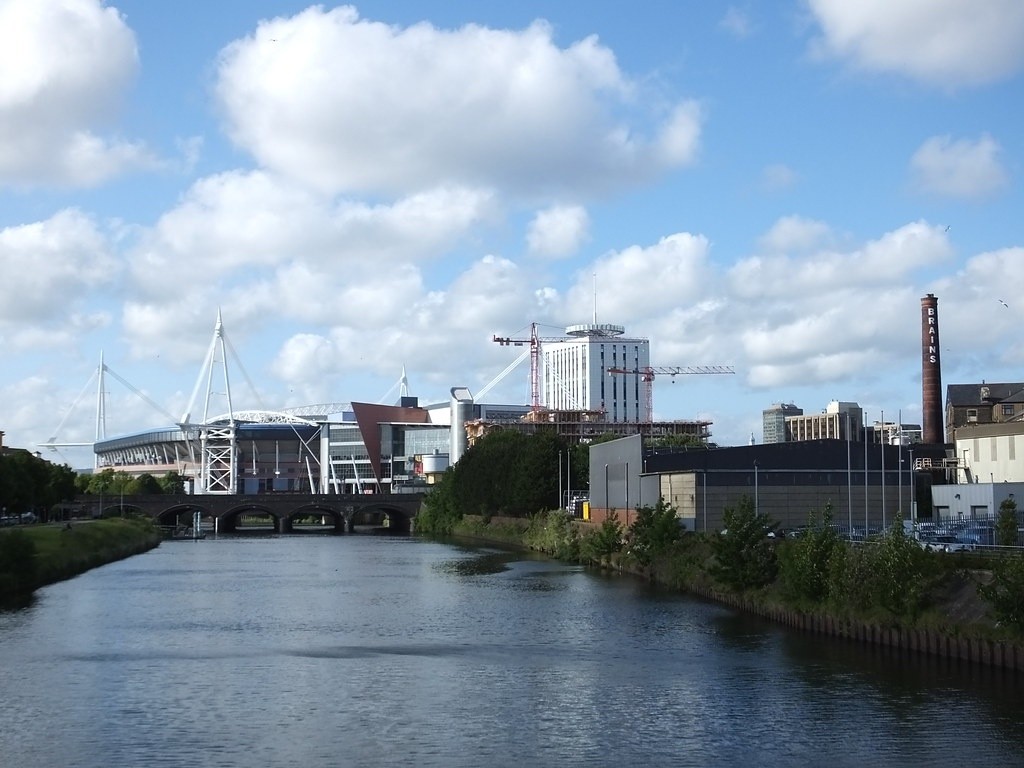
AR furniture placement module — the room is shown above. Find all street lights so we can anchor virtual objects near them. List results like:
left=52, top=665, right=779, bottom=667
left=121, top=483, right=126, bottom=515
left=99, top=482, right=110, bottom=519
left=753, top=456, right=760, bottom=517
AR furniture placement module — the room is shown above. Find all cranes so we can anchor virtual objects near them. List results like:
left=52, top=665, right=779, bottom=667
left=606, top=365, right=736, bottom=421
left=493, top=322, right=650, bottom=410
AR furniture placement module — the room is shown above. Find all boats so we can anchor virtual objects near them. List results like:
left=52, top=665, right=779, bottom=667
left=172, top=525, right=206, bottom=540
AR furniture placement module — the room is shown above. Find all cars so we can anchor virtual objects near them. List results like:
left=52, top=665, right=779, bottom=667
left=0, top=512, right=38, bottom=526
left=767, top=520, right=996, bottom=553
